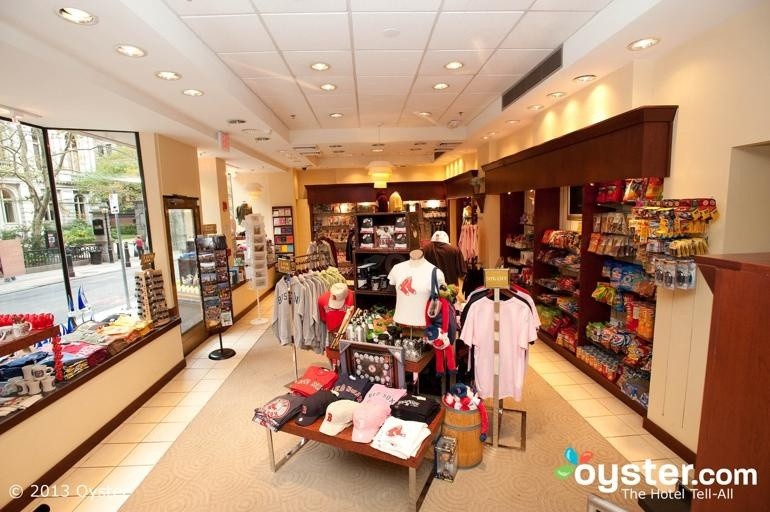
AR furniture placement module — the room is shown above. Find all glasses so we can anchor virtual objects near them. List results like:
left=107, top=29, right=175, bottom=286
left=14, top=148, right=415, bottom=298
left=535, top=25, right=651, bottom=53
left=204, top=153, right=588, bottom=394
left=134, top=273, right=171, bottom=325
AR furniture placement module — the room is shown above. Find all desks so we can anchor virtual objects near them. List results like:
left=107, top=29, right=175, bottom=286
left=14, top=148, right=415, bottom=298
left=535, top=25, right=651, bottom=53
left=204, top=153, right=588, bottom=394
left=263, top=385, right=446, bottom=511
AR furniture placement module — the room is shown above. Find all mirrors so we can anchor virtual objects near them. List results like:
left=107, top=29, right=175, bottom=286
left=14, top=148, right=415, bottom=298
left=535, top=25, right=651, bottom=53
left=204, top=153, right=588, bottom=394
left=165, top=194, right=205, bottom=335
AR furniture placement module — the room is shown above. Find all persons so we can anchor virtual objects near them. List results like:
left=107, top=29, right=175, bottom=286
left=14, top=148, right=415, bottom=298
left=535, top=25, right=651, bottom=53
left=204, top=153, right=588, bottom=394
left=133, top=235, right=144, bottom=259
left=387, top=250, right=448, bottom=327
left=419, top=230, right=466, bottom=302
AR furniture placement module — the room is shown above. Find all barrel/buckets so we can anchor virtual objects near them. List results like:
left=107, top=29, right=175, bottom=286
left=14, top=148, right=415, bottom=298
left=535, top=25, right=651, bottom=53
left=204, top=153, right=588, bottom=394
left=441, top=403, right=482, bottom=469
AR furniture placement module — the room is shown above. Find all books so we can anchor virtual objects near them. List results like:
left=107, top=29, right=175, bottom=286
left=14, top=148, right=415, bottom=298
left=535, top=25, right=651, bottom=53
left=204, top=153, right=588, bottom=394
left=272, top=207, right=294, bottom=272
left=197, top=234, right=233, bottom=330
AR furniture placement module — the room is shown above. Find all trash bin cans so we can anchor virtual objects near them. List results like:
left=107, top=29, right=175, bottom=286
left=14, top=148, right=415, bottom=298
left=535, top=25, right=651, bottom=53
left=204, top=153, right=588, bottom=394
left=90, top=250, right=101, bottom=264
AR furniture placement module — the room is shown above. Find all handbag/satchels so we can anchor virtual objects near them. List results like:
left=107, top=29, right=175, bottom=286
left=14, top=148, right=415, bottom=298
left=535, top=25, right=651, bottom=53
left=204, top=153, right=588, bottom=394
left=426, top=266, right=457, bottom=345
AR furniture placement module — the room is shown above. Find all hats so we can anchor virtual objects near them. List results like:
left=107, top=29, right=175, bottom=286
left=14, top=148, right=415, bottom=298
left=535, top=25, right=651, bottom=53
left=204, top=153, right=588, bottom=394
left=294, top=390, right=338, bottom=427
left=351, top=399, right=392, bottom=444
left=430, top=230, right=453, bottom=245
left=319, top=399, right=359, bottom=438
left=328, top=281, right=349, bottom=310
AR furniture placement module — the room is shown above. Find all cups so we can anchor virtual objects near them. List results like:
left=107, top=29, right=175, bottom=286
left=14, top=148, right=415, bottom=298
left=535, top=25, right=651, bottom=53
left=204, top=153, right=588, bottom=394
left=357, top=262, right=389, bottom=291
left=9, top=364, right=56, bottom=395
left=0, top=322, right=32, bottom=339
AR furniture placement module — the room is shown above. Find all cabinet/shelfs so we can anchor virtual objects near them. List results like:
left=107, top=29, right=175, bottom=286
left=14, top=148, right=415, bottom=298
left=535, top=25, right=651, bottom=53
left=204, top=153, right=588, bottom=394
left=504, top=242, right=582, bottom=356
left=313, top=213, right=352, bottom=253
left=354, top=212, right=421, bottom=315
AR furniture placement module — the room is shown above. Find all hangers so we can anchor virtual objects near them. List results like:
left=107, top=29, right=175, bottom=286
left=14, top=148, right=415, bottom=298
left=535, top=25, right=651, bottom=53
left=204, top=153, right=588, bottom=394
left=281, top=254, right=329, bottom=281
left=487, top=286, right=513, bottom=301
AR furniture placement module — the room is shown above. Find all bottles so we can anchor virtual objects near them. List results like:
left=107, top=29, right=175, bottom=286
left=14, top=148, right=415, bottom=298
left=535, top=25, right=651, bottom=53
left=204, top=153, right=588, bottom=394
left=347, top=311, right=389, bottom=346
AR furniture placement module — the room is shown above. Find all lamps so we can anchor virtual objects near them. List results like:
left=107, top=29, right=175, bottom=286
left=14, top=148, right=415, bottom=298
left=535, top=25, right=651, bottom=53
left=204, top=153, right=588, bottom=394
left=365, top=123, right=396, bottom=188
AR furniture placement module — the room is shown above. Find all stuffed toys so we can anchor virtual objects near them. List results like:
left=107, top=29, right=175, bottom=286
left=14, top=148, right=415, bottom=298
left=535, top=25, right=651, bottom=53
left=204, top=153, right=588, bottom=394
left=439, top=442, right=457, bottom=475
